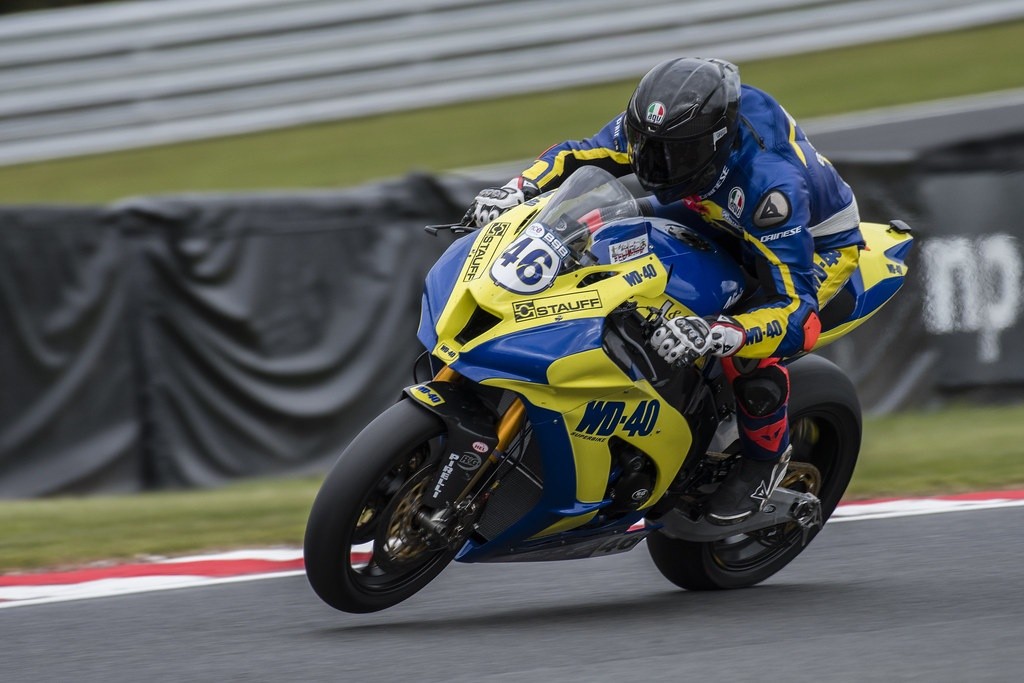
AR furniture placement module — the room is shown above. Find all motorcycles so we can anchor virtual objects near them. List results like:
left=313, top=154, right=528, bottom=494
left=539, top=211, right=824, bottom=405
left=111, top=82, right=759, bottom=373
left=301, top=163, right=913, bottom=614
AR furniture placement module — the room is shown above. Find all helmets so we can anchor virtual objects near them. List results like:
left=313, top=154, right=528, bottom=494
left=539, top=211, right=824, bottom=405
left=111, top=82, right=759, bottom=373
left=627, top=57, right=742, bottom=206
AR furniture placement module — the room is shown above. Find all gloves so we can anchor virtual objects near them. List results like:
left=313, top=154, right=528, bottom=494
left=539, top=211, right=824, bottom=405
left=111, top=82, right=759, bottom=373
left=460, top=176, right=542, bottom=229
left=650, top=314, right=746, bottom=369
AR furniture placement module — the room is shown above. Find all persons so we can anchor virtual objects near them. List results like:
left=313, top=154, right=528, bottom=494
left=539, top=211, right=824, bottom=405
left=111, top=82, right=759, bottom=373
left=458, top=56, right=867, bottom=527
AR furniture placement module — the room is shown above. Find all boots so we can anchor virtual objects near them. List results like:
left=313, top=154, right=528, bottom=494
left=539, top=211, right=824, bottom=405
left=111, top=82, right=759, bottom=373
left=704, top=401, right=792, bottom=526
